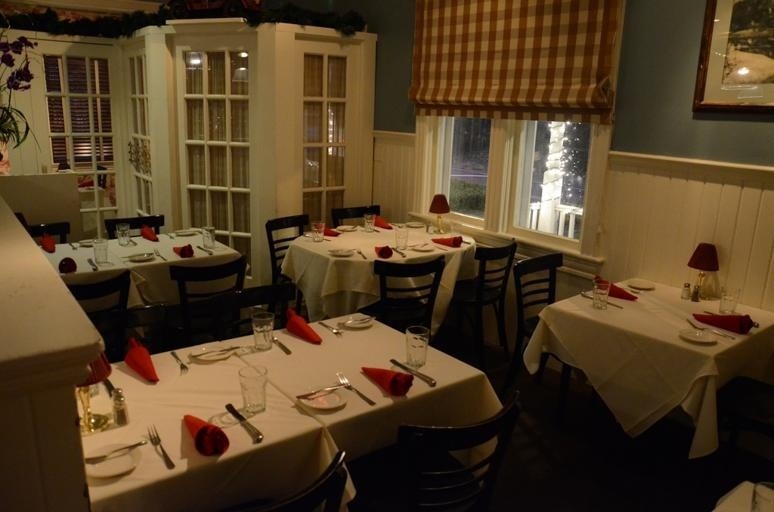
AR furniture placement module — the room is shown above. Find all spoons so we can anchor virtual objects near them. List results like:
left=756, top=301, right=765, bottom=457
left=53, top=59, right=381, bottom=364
left=68, top=241, right=77, bottom=250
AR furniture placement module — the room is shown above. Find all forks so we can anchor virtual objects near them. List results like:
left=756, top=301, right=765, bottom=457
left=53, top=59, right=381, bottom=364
left=172, top=351, right=189, bottom=372
left=319, top=321, right=344, bottom=334
left=147, top=424, right=175, bottom=469
left=336, top=372, right=376, bottom=406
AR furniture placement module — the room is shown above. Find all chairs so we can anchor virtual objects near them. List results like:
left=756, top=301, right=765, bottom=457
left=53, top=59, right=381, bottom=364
left=274, top=449, right=348, bottom=512
left=396, top=391, right=520, bottom=512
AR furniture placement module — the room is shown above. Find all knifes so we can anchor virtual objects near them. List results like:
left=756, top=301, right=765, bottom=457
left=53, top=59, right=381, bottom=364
left=85, top=440, right=147, bottom=462
left=87, top=259, right=99, bottom=271
left=271, top=334, right=291, bottom=354
left=581, top=291, right=623, bottom=310
left=303, top=233, right=332, bottom=241
left=103, top=376, right=116, bottom=398
left=295, top=385, right=344, bottom=400
left=225, top=404, right=264, bottom=442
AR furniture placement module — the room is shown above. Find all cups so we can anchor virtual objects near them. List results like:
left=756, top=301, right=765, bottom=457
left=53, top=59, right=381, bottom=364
left=239, top=365, right=268, bottom=413
left=251, top=314, right=274, bottom=351
left=237, top=313, right=437, bottom=416
left=719, top=286, right=741, bottom=315
left=93, top=222, right=216, bottom=265
left=592, top=279, right=612, bottom=309
left=311, top=214, right=409, bottom=250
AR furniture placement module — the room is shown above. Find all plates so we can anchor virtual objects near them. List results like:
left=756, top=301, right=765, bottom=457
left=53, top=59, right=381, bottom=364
left=78, top=229, right=197, bottom=263
left=678, top=327, right=718, bottom=345
left=327, top=221, right=434, bottom=260
left=300, top=385, right=348, bottom=410
left=85, top=443, right=141, bottom=478
left=628, top=279, right=655, bottom=289
left=336, top=315, right=374, bottom=330
left=190, top=342, right=234, bottom=361
left=79, top=413, right=110, bottom=437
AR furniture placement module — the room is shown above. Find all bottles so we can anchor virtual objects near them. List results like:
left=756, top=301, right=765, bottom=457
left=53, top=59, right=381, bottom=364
left=681, top=283, right=700, bottom=302
left=111, top=388, right=130, bottom=428
